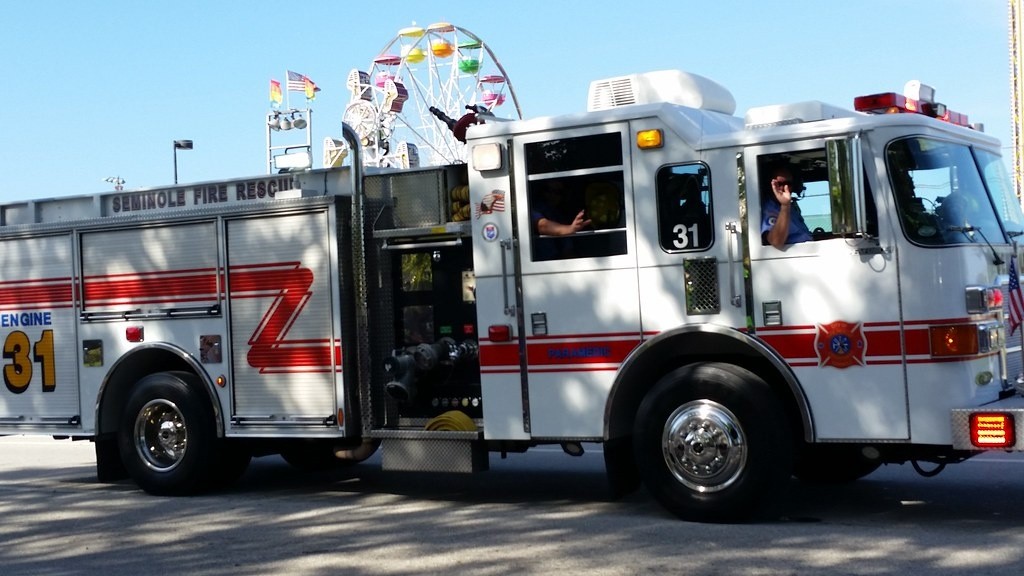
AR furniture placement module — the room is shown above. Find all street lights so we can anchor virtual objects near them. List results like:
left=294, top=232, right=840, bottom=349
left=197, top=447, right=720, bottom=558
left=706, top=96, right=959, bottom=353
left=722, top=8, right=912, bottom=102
left=172, top=138, right=194, bottom=184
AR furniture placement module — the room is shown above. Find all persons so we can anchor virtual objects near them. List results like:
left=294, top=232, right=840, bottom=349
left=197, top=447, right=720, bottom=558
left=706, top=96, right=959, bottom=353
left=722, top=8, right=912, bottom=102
left=529, top=170, right=592, bottom=261
left=760, top=166, right=814, bottom=249
left=658, top=175, right=707, bottom=248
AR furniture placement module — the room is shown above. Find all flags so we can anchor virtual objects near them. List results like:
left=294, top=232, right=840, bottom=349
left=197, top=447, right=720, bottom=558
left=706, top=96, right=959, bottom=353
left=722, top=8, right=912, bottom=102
left=1008, top=261, right=1024, bottom=336
left=288, top=70, right=321, bottom=91
left=270, top=80, right=283, bottom=105
left=304, top=77, right=315, bottom=99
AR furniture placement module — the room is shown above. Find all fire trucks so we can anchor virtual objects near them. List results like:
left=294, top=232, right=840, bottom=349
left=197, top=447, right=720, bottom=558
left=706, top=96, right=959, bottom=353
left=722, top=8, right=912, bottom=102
left=0, top=70, right=1024, bottom=525
